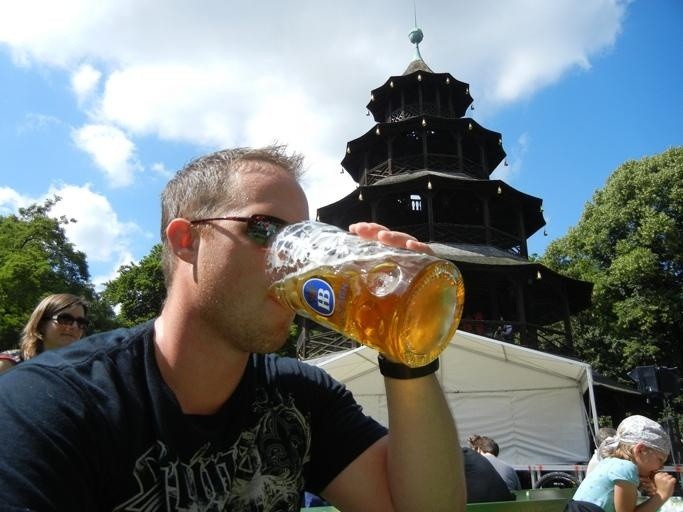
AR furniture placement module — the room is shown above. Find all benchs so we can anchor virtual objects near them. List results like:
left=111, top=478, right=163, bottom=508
left=300, top=484, right=581, bottom=512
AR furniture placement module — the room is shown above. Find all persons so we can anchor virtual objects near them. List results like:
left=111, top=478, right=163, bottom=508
left=561, top=414, right=677, bottom=511
left=582, top=425, right=618, bottom=477
left=0, top=295, right=87, bottom=372
left=466, top=433, right=521, bottom=490
left=0, top=142, right=468, bottom=512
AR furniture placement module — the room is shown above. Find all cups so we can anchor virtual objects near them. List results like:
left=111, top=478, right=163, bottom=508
left=262, top=217, right=464, bottom=370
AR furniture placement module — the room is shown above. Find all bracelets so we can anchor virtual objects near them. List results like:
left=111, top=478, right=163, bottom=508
left=376, top=352, right=439, bottom=381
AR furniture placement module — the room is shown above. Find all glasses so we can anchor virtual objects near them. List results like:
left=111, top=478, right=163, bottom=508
left=47, top=311, right=90, bottom=330
left=191, top=213, right=287, bottom=248
